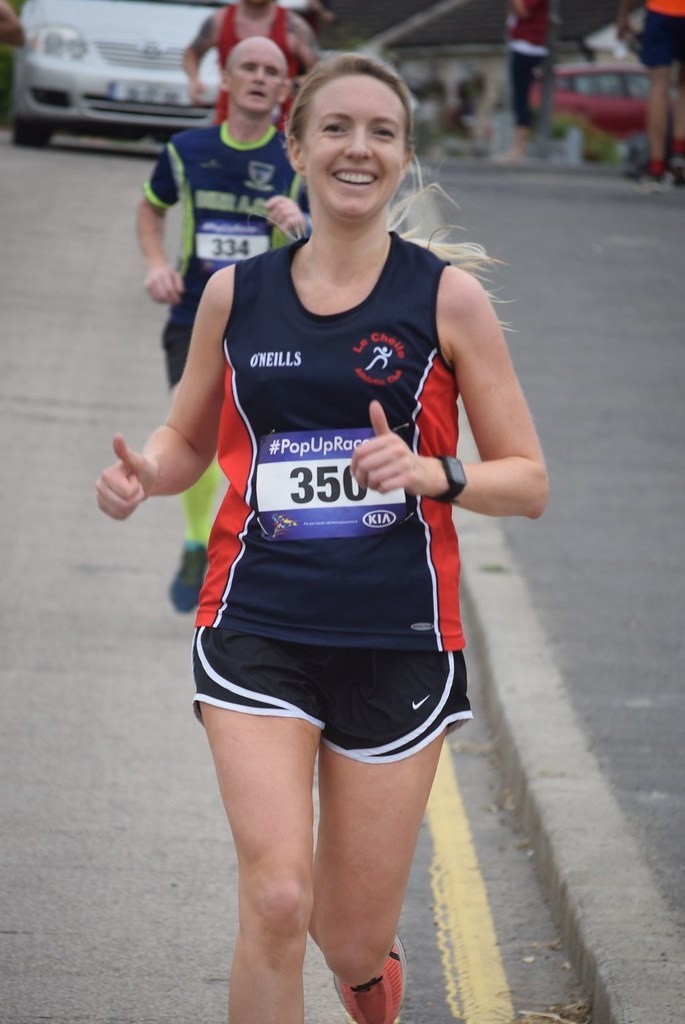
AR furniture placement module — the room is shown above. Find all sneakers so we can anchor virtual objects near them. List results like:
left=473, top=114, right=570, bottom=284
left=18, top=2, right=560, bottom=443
left=333, top=930, right=406, bottom=1024
left=170, top=542, right=208, bottom=613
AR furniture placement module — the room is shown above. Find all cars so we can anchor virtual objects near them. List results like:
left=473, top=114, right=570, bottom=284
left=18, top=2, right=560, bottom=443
left=10, top=0, right=237, bottom=148
left=527, top=62, right=684, bottom=180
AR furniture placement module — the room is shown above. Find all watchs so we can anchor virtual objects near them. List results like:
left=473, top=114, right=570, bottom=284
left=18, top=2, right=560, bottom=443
left=427, top=453, right=467, bottom=502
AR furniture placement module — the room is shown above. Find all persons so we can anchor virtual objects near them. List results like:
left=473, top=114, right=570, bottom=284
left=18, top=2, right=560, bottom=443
left=96, top=50, right=551, bottom=1024
left=0, top=0, right=26, bottom=119
left=183, top=0, right=315, bottom=134
left=505, top=0, right=552, bottom=152
left=134, top=35, right=310, bottom=612
left=616, top=0, right=685, bottom=186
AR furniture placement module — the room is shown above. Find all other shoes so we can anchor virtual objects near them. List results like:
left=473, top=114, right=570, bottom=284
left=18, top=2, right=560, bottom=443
left=671, top=155, right=685, bottom=183
